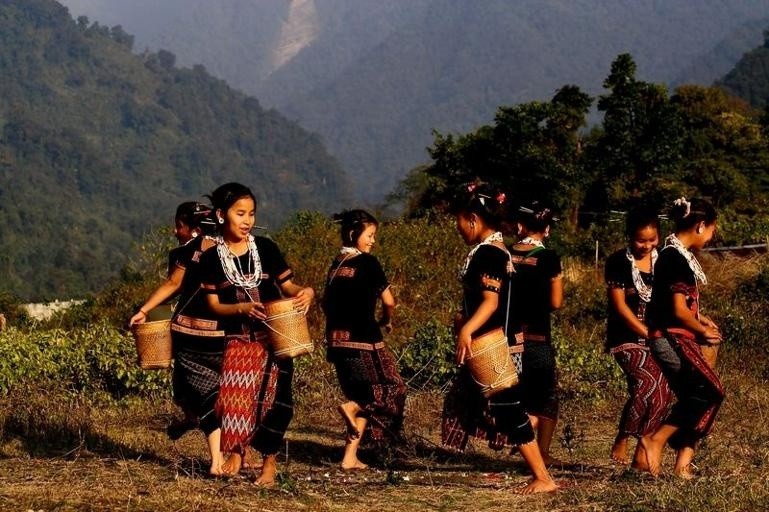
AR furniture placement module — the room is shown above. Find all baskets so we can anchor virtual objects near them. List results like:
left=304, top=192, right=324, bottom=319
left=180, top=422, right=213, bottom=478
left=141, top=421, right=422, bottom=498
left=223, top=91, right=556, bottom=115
left=465, top=327, right=519, bottom=398
left=262, top=296, right=315, bottom=359
left=700, top=345, right=718, bottom=370
left=132, top=319, right=172, bottom=370
